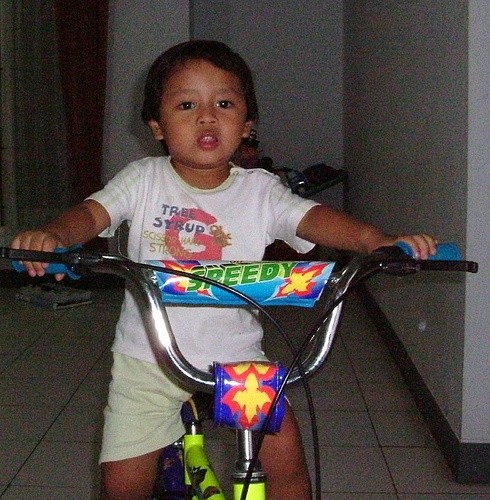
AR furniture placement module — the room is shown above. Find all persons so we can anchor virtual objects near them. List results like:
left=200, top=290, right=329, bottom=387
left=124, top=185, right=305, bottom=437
left=6, top=41, right=437, bottom=500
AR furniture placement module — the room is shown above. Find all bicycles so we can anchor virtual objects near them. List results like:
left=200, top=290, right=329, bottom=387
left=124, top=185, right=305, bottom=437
left=0, top=232, right=478, bottom=500
